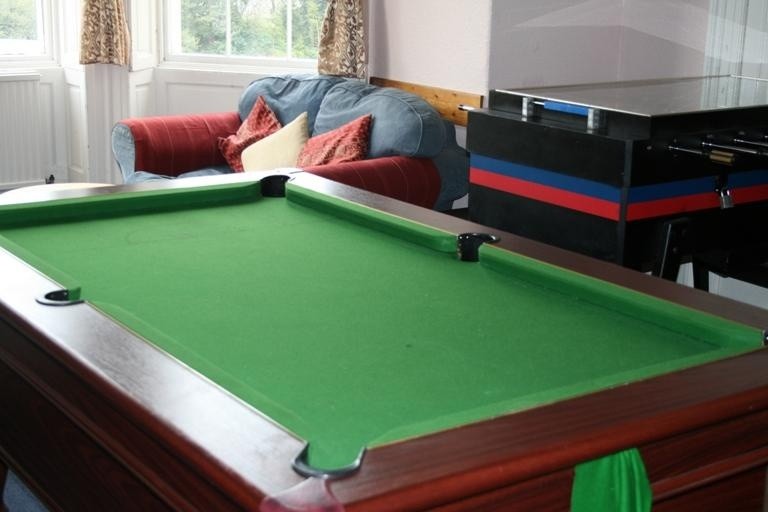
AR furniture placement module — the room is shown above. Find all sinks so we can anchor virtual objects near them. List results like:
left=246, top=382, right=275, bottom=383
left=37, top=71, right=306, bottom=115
left=110, top=73, right=468, bottom=211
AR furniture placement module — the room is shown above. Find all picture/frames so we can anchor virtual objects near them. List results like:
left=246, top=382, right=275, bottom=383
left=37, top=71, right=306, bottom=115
left=0, top=170, right=768, bottom=512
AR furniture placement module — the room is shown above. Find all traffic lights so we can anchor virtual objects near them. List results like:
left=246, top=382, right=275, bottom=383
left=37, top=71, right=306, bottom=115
left=216, top=95, right=373, bottom=175
